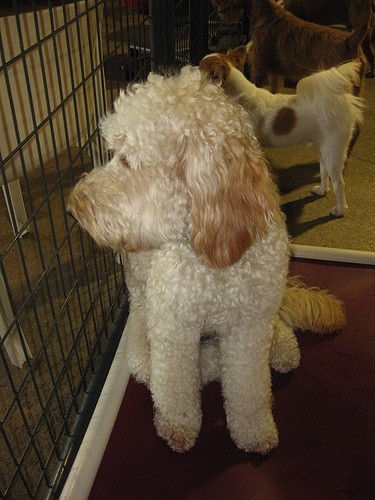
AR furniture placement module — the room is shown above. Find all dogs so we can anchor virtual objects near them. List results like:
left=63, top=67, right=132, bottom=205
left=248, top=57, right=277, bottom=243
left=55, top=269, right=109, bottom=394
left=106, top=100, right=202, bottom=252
left=195, top=45, right=368, bottom=216
left=217, top=0, right=372, bottom=158
left=65, top=65, right=347, bottom=455
